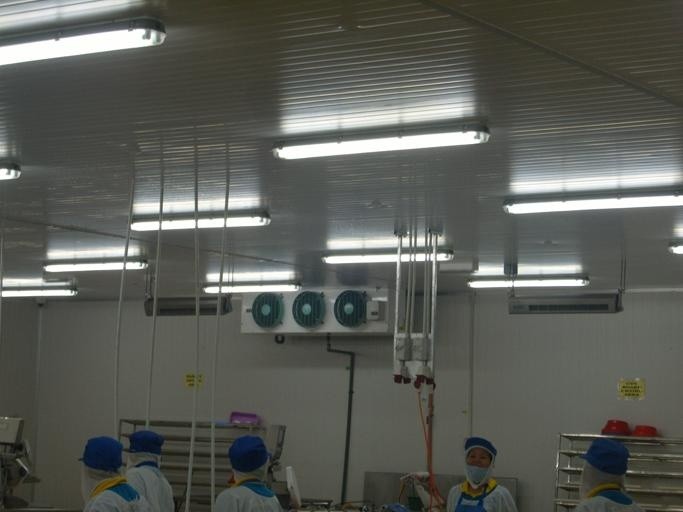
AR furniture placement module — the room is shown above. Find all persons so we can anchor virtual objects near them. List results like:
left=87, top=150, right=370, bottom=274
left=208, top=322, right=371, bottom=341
left=214, top=435, right=286, bottom=512
left=122, top=431, right=175, bottom=512
left=77, top=435, right=148, bottom=512
left=568, top=438, right=644, bottom=512
left=447, top=436, right=518, bottom=512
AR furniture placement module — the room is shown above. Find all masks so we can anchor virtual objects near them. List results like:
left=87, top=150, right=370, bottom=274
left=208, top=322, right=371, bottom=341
left=464, top=464, right=489, bottom=483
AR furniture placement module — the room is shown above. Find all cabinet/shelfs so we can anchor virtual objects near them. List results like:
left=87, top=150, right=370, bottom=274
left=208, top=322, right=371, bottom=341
left=552, top=430, right=682, bottom=512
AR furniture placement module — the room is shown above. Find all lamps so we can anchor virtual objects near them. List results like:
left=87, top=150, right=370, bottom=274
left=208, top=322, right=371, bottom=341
left=271, top=123, right=490, bottom=159
left=468, top=277, right=588, bottom=289
left=132, top=213, right=272, bottom=233
left=1, top=286, right=76, bottom=298
left=323, top=250, right=454, bottom=264
left=44, top=260, right=149, bottom=272
left=502, top=191, right=682, bottom=214
left=1, top=16, right=166, bottom=65
left=203, top=284, right=301, bottom=294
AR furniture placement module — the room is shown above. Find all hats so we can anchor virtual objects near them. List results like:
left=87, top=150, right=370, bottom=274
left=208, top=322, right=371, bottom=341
left=577, top=438, right=629, bottom=475
left=77, top=436, right=123, bottom=471
left=228, top=436, right=271, bottom=472
left=124, top=430, right=164, bottom=454
left=465, top=437, right=497, bottom=457
